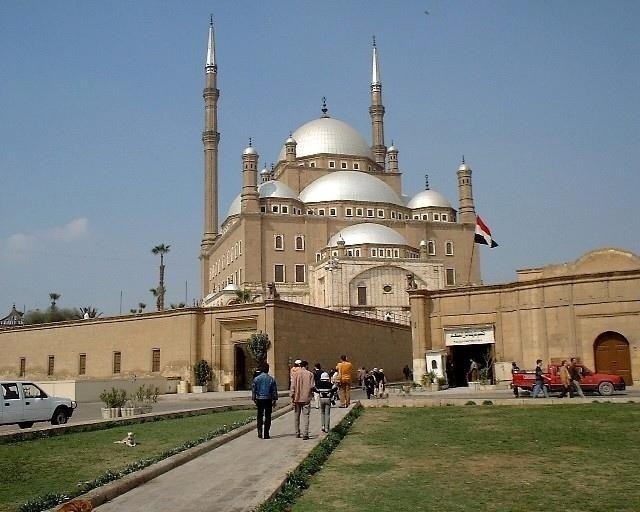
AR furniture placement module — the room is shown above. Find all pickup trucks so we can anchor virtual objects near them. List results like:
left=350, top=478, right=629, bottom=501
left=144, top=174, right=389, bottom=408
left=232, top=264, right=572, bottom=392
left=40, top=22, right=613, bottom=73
left=511, top=363, right=626, bottom=398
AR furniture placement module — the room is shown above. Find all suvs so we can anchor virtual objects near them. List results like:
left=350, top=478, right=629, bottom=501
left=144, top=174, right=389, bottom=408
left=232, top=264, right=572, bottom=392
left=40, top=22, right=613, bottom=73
left=0, top=381, right=77, bottom=428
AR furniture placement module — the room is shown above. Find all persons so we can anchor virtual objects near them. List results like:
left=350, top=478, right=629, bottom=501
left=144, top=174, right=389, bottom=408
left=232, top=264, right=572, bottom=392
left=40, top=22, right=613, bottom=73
left=558, top=359, right=574, bottom=399
left=569, top=357, right=586, bottom=397
left=402, top=363, right=409, bottom=379
left=311, top=370, right=332, bottom=433
left=290, top=355, right=389, bottom=410
left=511, top=361, right=520, bottom=397
left=290, top=360, right=312, bottom=440
left=252, top=362, right=276, bottom=439
left=446, top=357, right=478, bottom=388
left=533, top=359, right=552, bottom=398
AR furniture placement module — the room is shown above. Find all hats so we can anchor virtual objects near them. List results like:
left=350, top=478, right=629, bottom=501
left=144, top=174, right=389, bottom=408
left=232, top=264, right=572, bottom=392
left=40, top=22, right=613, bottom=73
left=321, top=372, right=329, bottom=380
left=295, top=360, right=302, bottom=364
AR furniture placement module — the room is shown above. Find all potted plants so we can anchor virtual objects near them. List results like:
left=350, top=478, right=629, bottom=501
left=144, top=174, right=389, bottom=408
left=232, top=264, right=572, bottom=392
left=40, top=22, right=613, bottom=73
left=96, top=380, right=161, bottom=421
left=189, top=358, right=212, bottom=396
left=400, top=368, right=447, bottom=395
left=464, top=344, right=498, bottom=394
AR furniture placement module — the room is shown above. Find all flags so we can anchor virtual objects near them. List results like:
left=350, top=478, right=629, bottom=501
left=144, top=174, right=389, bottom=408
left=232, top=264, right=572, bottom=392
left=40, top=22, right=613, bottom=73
left=473, top=215, right=500, bottom=249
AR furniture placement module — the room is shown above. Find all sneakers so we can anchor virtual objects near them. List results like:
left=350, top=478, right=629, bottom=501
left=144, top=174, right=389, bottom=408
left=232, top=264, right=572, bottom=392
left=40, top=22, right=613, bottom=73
left=315, top=394, right=351, bottom=408
left=257, top=425, right=328, bottom=440
left=514, top=395, right=586, bottom=398
